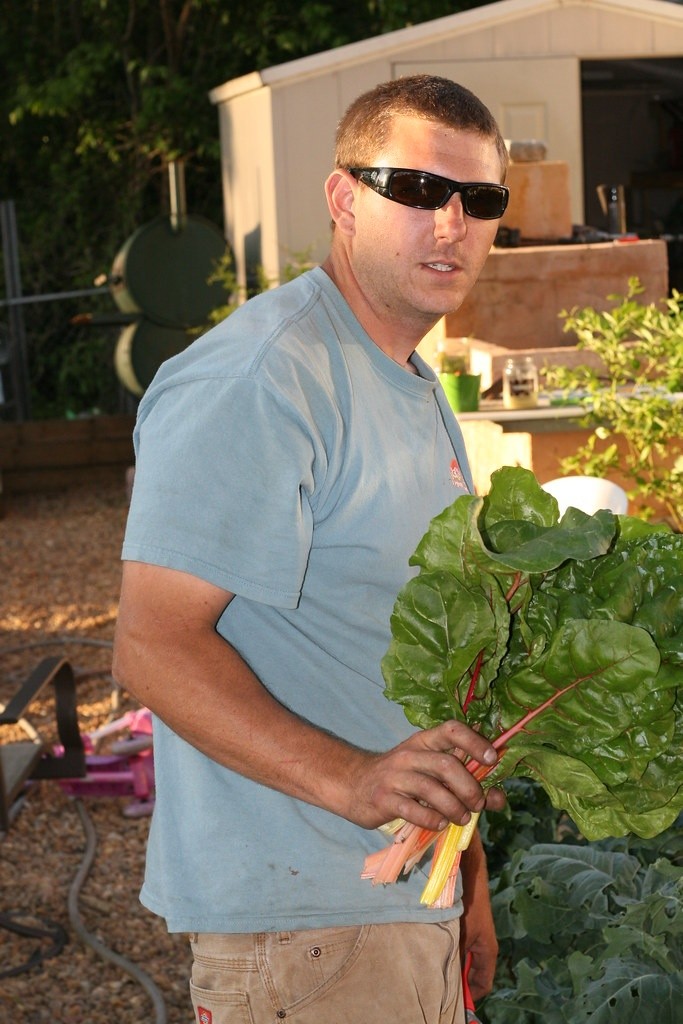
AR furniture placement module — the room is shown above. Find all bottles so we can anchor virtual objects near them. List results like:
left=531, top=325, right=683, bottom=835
left=436, top=337, right=471, bottom=375
left=502, top=357, right=540, bottom=410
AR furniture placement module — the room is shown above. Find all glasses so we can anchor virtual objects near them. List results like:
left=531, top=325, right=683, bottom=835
left=348, top=166, right=510, bottom=221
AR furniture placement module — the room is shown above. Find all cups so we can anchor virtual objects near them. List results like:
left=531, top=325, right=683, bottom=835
left=439, top=373, right=482, bottom=412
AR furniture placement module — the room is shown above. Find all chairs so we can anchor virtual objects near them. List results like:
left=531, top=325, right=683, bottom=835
left=0, top=655, right=87, bottom=979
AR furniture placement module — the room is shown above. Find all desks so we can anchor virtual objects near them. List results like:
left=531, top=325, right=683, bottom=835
left=448, top=385, right=683, bottom=529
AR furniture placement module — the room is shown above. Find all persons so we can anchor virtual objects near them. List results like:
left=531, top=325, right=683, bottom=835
left=112, top=75, right=510, bottom=1023
